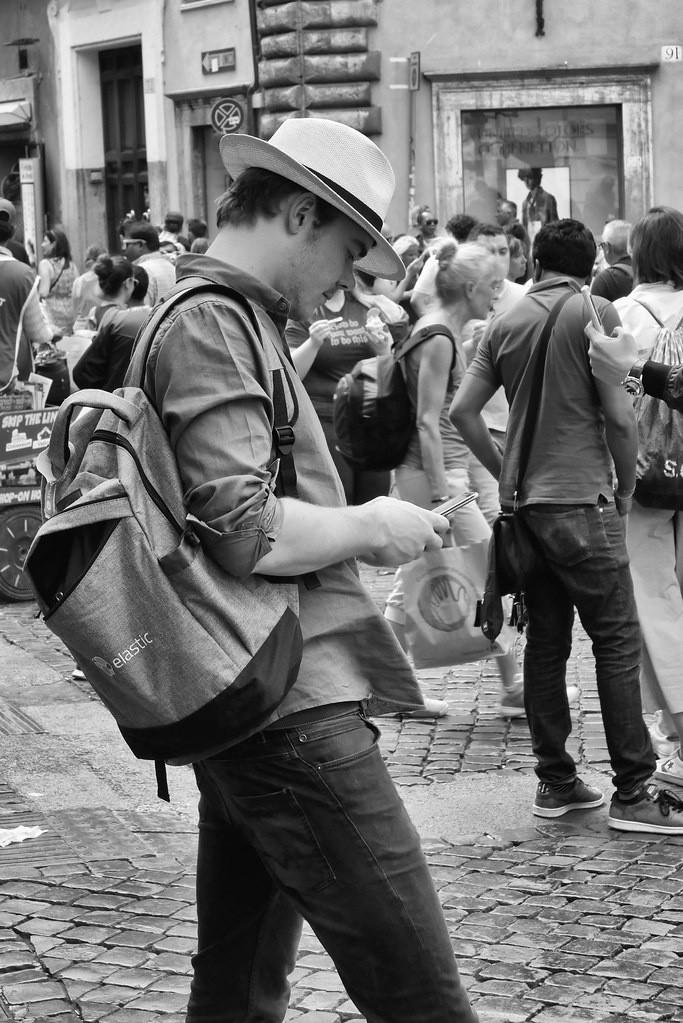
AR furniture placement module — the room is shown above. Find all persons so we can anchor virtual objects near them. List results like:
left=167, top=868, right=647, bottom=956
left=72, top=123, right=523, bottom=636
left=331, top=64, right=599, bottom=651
left=447, top=217, right=683, bottom=832
left=285, top=206, right=530, bottom=506
left=517, top=166, right=560, bottom=228
left=1, top=196, right=211, bottom=395
left=584, top=207, right=683, bottom=787
left=381, top=237, right=581, bottom=719
left=122, top=119, right=475, bottom=1023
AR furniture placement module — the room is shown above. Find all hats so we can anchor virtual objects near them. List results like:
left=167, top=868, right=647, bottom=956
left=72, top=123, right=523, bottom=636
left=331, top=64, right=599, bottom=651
left=220, top=117, right=407, bottom=282
left=0, top=198, right=17, bottom=224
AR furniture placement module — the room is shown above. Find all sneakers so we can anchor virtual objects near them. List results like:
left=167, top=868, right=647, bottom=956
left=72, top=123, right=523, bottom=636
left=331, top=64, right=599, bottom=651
left=652, top=744, right=683, bottom=786
left=606, top=784, right=683, bottom=834
left=648, top=709, right=682, bottom=759
left=532, top=775, right=605, bottom=817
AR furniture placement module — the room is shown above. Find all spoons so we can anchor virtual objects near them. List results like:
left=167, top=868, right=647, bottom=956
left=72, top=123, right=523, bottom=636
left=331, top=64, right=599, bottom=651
left=327, top=317, right=343, bottom=323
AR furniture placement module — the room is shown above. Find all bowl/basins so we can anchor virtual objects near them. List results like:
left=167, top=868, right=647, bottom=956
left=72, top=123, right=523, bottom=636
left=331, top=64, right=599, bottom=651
left=366, top=324, right=384, bottom=340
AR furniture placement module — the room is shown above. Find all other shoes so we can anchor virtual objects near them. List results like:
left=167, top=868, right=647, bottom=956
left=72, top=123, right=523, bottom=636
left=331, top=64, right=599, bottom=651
left=407, top=695, right=448, bottom=718
left=499, top=685, right=581, bottom=718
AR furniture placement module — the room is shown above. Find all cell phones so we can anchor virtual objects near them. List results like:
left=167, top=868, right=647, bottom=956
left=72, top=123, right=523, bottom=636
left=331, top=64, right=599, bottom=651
left=581, top=285, right=605, bottom=336
left=430, top=491, right=478, bottom=516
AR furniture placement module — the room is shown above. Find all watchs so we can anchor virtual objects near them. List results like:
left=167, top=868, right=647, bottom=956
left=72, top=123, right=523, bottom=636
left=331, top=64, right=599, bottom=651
left=625, top=359, right=647, bottom=398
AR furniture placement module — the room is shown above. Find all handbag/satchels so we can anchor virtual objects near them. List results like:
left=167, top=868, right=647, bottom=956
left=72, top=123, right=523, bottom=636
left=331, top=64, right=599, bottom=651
left=71, top=307, right=127, bottom=390
left=37, top=298, right=63, bottom=343
left=475, top=518, right=529, bottom=643
left=399, top=538, right=516, bottom=668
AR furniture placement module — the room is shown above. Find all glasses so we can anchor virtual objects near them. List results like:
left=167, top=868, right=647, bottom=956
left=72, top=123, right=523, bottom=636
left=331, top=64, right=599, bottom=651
left=596, top=242, right=609, bottom=251
left=123, top=238, right=147, bottom=253
left=480, top=280, right=505, bottom=296
left=421, top=219, right=438, bottom=227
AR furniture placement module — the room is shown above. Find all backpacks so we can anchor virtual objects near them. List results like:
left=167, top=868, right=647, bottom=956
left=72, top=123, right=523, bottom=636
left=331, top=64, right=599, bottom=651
left=622, top=295, right=683, bottom=510
left=23, top=283, right=296, bottom=767
left=334, top=324, right=457, bottom=473
